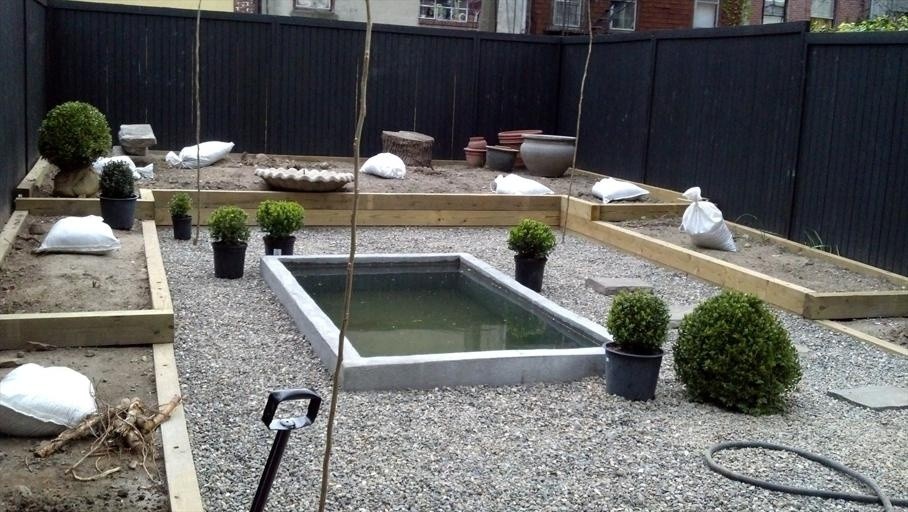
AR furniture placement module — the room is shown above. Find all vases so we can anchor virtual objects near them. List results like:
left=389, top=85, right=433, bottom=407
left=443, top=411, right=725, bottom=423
left=465, top=127, right=542, bottom=172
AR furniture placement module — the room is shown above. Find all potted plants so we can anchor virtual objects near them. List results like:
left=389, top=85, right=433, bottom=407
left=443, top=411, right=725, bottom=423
left=168, top=191, right=193, bottom=240
left=508, top=218, right=557, bottom=291
left=99, top=160, right=137, bottom=231
left=208, top=204, right=249, bottom=278
left=256, top=198, right=305, bottom=256
left=604, top=292, right=668, bottom=401
